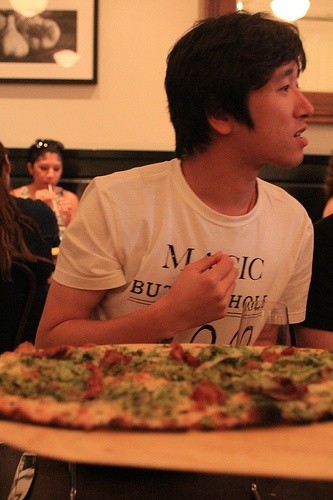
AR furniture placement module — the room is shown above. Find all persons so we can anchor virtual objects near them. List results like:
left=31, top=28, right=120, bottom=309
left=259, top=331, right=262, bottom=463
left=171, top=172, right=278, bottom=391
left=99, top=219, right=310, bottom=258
left=9, top=138, right=79, bottom=227
left=35, top=9, right=314, bottom=348
left=0, top=142, right=60, bottom=354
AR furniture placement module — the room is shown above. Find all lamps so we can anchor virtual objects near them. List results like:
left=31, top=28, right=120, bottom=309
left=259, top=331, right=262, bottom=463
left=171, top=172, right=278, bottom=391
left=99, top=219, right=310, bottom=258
left=53, top=49, right=80, bottom=68
left=10, top=0, right=48, bottom=18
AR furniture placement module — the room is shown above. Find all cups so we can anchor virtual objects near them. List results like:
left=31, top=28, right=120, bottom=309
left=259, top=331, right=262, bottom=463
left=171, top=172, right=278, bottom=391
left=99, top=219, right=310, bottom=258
left=49, top=223, right=66, bottom=256
left=236, top=301, right=291, bottom=347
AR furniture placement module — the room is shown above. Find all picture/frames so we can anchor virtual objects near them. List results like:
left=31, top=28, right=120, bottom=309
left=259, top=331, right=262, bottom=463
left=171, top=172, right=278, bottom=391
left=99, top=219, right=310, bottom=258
left=0, top=0, right=98, bottom=83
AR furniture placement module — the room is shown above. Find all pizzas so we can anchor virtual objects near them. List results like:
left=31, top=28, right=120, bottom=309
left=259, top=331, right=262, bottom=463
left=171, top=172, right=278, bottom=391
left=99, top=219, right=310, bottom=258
left=0, top=342, right=333, bottom=430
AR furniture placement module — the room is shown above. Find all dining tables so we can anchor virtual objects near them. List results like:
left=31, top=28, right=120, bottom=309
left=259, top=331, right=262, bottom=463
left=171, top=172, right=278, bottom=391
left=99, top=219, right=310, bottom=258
left=0, top=342, right=333, bottom=500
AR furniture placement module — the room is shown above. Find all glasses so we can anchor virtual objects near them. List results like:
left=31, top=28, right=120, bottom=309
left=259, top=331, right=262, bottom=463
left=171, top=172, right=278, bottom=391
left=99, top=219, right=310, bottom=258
left=29, top=139, right=64, bottom=155
